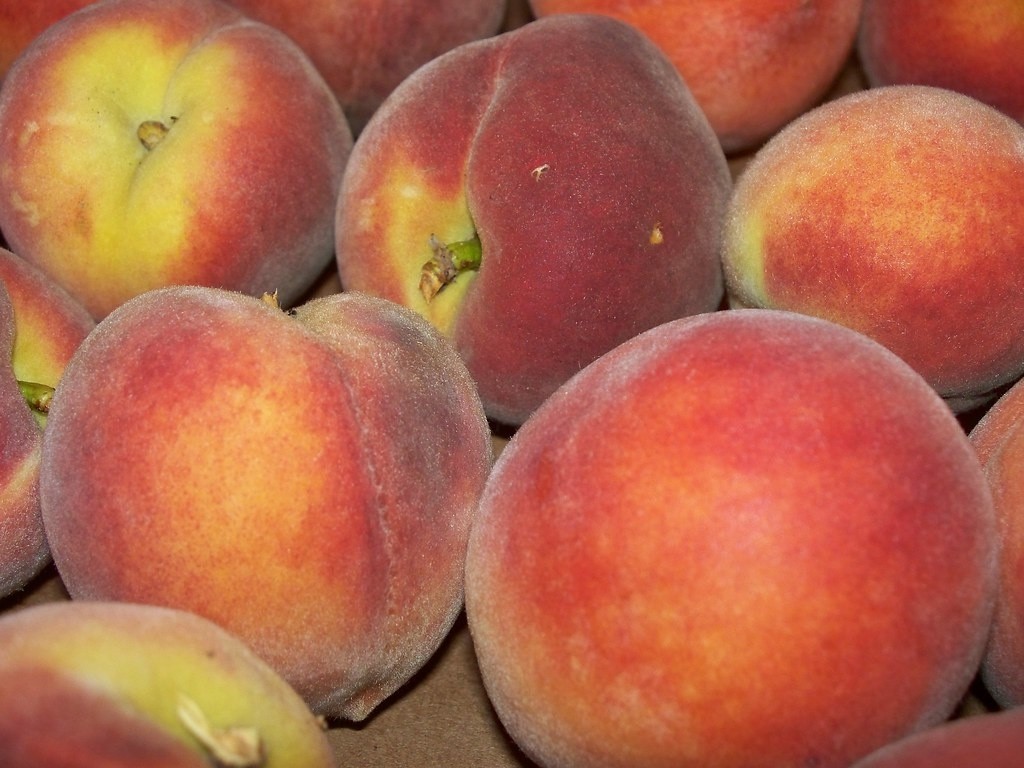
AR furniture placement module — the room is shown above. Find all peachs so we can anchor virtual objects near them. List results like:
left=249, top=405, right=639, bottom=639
left=0, top=0, right=1024, bottom=768
